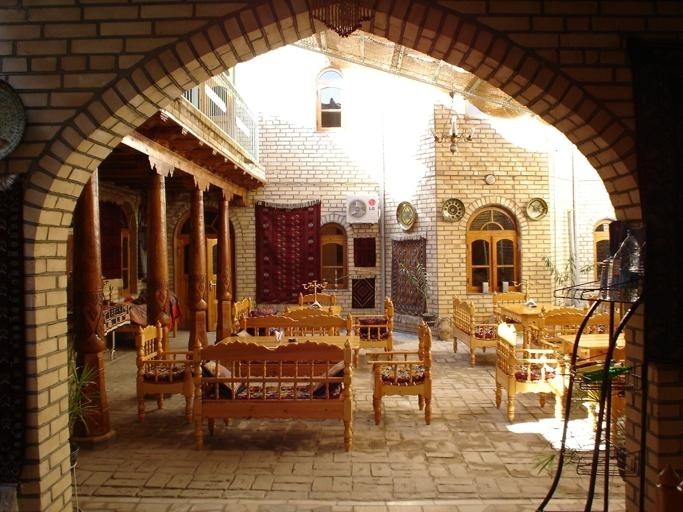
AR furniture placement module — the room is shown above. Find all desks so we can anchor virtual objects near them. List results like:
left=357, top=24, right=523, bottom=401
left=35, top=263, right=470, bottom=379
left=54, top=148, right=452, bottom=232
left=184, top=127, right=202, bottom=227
left=498, top=302, right=570, bottom=360
left=216, top=335, right=362, bottom=376
left=559, top=334, right=623, bottom=363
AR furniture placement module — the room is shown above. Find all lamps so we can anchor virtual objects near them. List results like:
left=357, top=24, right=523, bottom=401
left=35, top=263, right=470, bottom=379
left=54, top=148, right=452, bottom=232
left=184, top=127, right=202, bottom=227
left=430, top=91, right=475, bottom=154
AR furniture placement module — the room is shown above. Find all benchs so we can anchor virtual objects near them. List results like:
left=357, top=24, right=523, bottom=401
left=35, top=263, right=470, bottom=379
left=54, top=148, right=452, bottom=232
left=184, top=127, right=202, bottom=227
left=540, top=312, right=623, bottom=349
left=192, top=338, right=353, bottom=452
left=366, top=321, right=432, bottom=424
left=232, top=294, right=347, bottom=338
left=495, top=323, right=567, bottom=424
left=139, top=323, right=194, bottom=420
left=451, top=296, right=501, bottom=365
left=492, top=295, right=530, bottom=322
left=354, top=295, right=394, bottom=367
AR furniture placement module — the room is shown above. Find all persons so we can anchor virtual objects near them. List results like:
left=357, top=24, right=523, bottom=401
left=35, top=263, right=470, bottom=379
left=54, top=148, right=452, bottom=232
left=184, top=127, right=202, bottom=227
left=124, top=278, right=177, bottom=314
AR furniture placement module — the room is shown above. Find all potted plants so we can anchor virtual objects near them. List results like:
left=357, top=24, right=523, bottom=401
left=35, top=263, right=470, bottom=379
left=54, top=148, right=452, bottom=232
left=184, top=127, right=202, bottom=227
left=67, top=348, right=96, bottom=469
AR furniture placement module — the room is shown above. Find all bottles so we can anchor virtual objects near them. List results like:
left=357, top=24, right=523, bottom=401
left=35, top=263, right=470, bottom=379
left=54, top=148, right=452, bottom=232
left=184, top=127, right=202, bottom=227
left=581, top=364, right=633, bottom=384
left=598, top=228, right=642, bottom=301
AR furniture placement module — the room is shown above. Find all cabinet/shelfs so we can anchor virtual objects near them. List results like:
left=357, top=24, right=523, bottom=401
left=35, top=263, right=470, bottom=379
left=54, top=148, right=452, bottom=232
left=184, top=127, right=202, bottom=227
left=537, top=225, right=649, bottom=512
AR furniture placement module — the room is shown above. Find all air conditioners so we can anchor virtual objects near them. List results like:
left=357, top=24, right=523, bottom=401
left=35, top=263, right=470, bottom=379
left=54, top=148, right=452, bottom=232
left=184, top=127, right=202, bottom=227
left=346, top=193, right=378, bottom=223
